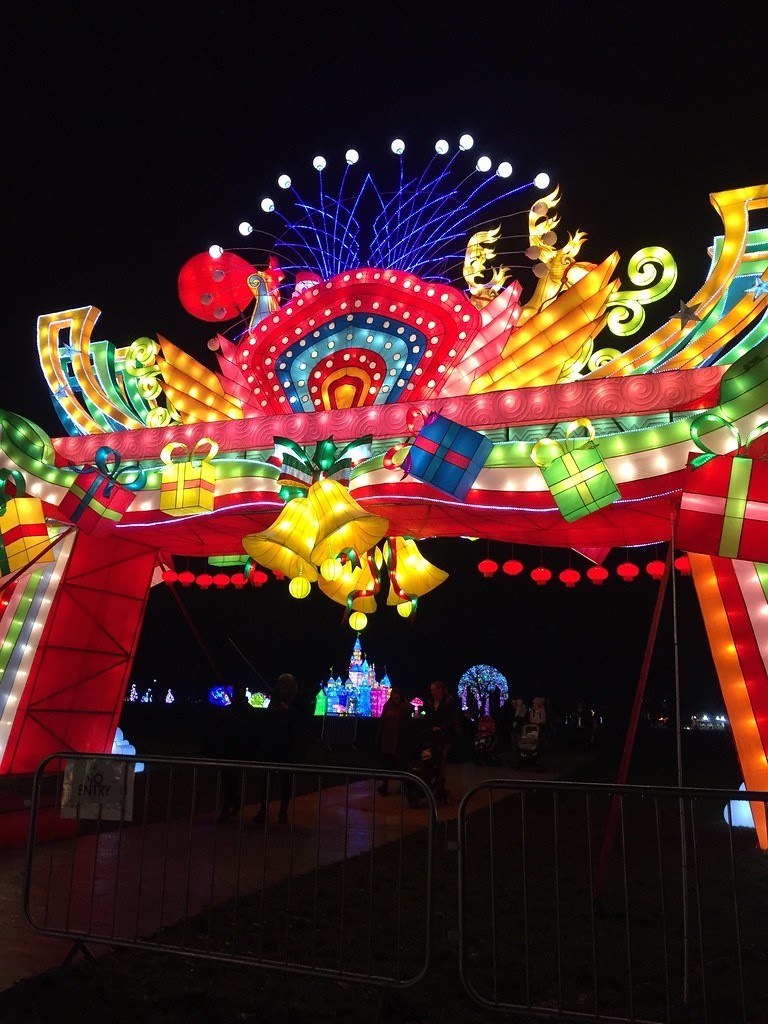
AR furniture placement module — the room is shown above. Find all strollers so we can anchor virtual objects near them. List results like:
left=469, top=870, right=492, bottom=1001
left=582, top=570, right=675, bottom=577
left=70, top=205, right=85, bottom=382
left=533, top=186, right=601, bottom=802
left=510, top=719, right=545, bottom=770
left=402, top=727, right=450, bottom=808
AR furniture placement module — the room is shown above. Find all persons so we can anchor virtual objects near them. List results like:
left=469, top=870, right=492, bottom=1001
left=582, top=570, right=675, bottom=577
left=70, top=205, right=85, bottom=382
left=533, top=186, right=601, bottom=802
left=406, top=681, right=459, bottom=808
left=203, top=677, right=249, bottom=825
left=255, top=674, right=307, bottom=828
left=472, top=694, right=551, bottom=768
left=380, top=690, right=405, bottom=795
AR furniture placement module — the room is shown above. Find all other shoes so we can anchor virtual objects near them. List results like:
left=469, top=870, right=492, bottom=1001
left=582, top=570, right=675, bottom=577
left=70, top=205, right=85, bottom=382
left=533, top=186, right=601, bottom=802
left=218, top=800, right=241, bottom=824
left=377, top=786, right=387, bottom=795
left=253, top=812, right=265, bottom=823
left=278, top=812, right=288, bottom=824
